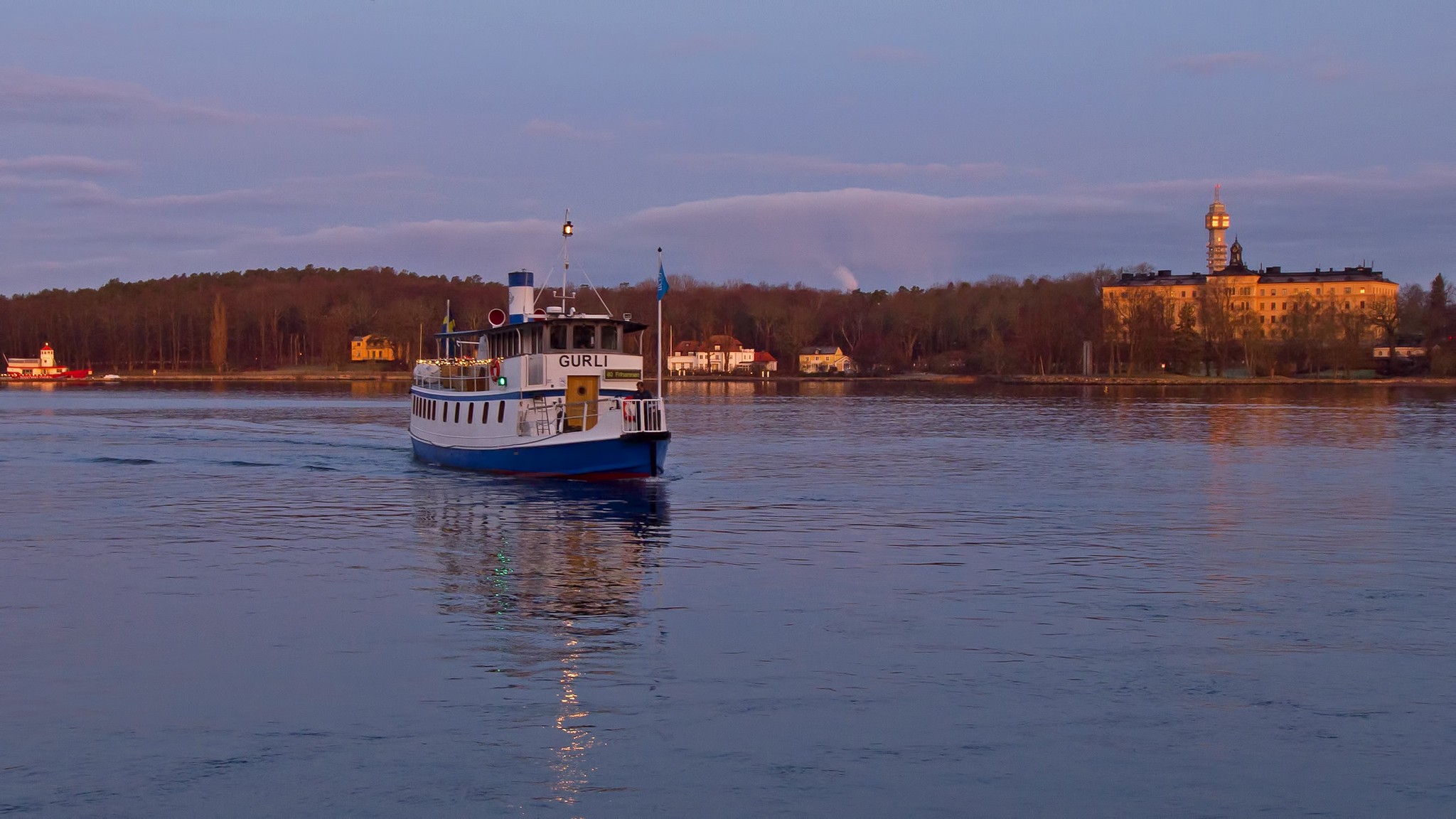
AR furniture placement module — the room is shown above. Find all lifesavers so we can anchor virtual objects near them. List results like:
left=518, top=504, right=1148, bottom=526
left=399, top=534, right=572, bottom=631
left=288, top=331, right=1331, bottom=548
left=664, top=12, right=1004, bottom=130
left=490, top=358, right=500, bottom=382
left=623, top=395, right=638, bottom=421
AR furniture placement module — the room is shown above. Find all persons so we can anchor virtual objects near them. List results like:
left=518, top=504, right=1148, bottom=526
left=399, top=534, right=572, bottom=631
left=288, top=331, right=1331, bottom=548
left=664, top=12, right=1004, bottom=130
left=632, top=381, right=654, bottom=431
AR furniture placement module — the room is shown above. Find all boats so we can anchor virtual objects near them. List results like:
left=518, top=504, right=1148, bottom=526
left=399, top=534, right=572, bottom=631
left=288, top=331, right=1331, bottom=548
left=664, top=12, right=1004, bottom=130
left=409, top=207, right=673, bottom=479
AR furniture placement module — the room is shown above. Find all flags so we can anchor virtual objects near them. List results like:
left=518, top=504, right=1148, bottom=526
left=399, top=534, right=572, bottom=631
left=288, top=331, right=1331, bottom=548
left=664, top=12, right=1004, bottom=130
left=656, top=255, right=670, bottom=302
left=441, top=304, right=459, bottom=359
